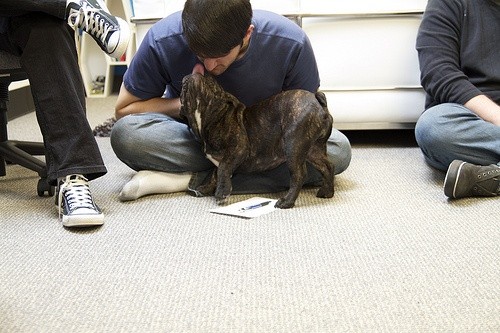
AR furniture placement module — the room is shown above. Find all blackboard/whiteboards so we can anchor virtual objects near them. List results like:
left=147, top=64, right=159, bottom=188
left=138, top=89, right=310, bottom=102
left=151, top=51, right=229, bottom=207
left=128, top=0, right=427, bottom=27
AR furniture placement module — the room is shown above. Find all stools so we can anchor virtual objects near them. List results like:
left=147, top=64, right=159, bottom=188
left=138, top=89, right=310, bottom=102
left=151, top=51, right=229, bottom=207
left=0, top=52, right=57, bottom=197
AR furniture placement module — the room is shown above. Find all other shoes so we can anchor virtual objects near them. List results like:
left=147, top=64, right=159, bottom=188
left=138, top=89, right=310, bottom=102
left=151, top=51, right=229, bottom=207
left=443, top=160, right=500, bottom=199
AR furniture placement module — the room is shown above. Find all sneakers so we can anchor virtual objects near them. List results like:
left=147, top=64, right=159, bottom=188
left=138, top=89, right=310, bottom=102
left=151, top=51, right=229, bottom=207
left=55, top=174, right=104, bottom=227
left=66, top=0, right=129, bottom=59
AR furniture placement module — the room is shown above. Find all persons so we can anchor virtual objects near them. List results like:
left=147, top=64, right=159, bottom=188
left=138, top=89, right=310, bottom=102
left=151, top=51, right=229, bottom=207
left=0, top=0, right=130, bottom=227
left=414, top=0, right=500, bottom=199
left=110, top=0, right=352, bottom=202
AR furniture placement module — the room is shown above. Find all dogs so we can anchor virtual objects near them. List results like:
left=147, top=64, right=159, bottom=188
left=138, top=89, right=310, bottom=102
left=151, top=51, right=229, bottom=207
left=180, top=73, right=335, bottom=209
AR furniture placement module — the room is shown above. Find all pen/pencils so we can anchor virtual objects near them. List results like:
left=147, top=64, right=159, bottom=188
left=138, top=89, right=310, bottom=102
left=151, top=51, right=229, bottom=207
left=239, top=200, right=271, bottom=212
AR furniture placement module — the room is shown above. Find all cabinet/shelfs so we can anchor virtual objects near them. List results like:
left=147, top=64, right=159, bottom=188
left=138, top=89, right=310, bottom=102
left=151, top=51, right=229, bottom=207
left=75, top=0, right=138, bottom=99
